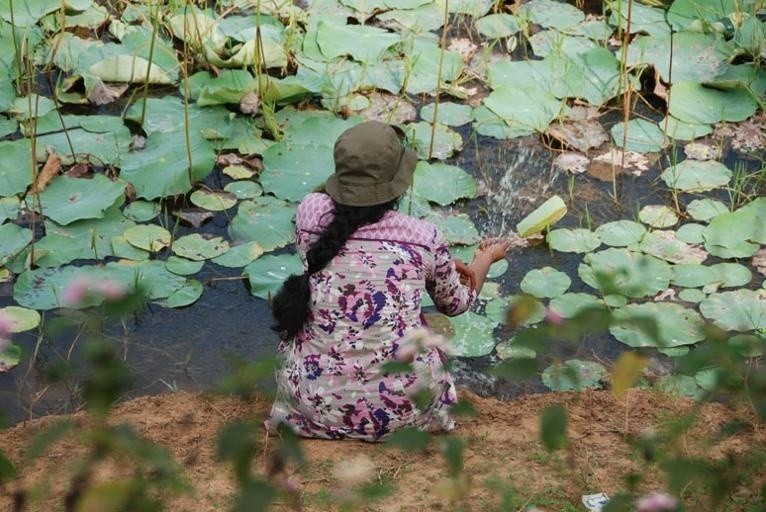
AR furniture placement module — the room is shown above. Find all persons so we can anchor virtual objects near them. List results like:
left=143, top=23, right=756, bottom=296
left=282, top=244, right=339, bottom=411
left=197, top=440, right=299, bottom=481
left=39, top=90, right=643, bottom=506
left=263, top=119, right=514, bottom=444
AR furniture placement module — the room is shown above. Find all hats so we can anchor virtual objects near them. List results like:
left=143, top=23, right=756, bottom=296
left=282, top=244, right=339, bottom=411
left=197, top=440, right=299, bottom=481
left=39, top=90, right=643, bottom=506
left=324, top=121, right=416, bottom=205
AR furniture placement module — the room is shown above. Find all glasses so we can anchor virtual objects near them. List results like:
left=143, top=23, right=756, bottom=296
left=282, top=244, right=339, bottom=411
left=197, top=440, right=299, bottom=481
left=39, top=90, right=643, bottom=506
left=391, top=123, right=408, bottom=182
